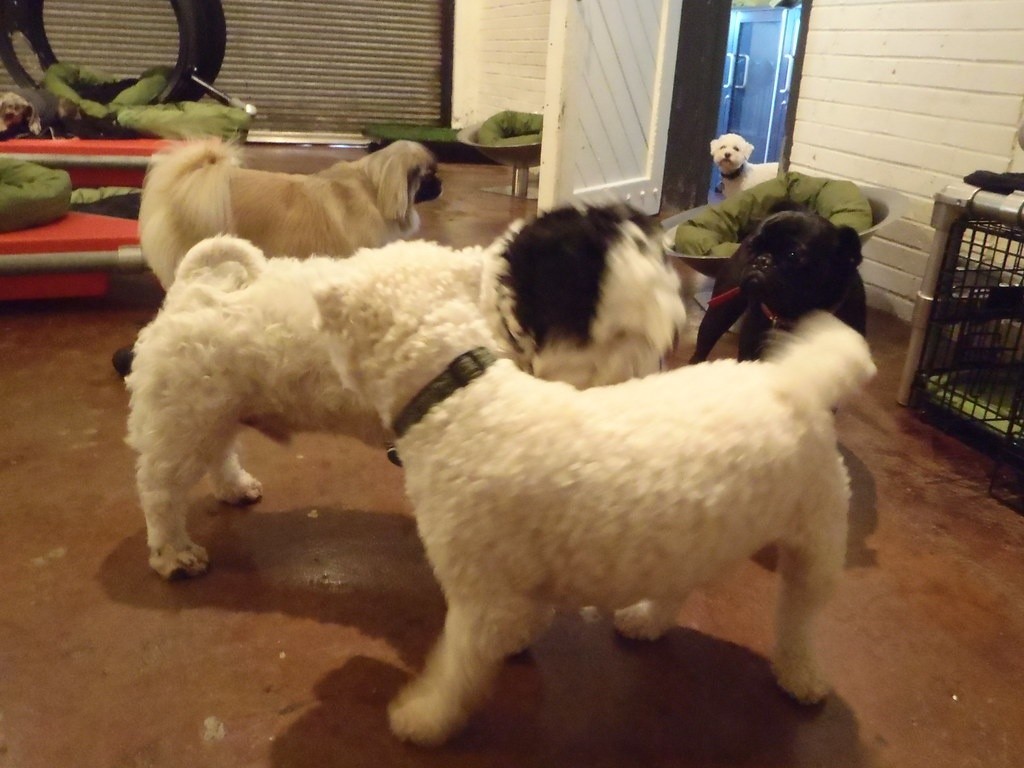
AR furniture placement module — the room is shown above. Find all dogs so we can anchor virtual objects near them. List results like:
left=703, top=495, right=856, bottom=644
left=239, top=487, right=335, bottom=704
left=123, top=195, right=681, bottom=584
left=0, top=92, right=43, bottom=135
left=711, top=134, right=781, bottom=199
left=310, top=245, right=881, bottom=744
left=684, top=211, right=867, bottom=365
left=141, top=135, right=443, bottom=296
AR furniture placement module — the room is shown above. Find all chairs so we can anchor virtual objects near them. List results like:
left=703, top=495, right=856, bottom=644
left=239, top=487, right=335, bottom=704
left=665, top=177, right=909, bottom=365
left=455, top=110, right=544, bottom=200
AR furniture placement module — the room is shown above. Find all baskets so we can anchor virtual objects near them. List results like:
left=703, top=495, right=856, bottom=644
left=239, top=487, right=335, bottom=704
left=912, top=215, right=1023, bottom=465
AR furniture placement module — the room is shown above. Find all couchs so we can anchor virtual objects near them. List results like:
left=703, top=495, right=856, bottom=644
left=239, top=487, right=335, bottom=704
left=0, top=139, right=180, bottom=305
